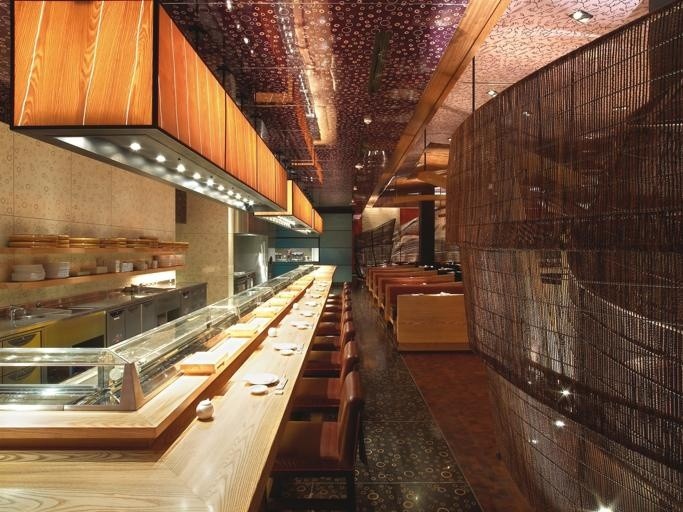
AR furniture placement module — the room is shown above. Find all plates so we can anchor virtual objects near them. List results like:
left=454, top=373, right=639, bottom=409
left=248, top=373, right=278, bottom=385
left=249, top=384, right=269, bottom=394
left=280, top=349, right=293, bottom=355
left=45, top=261, right=70, bottom=279
left=274, top=343, right=296, bottom=350
left=9, top=263, right=46, bottom=282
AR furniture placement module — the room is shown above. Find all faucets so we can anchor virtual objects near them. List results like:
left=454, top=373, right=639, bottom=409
left=9, top=305, right=24, bottom=320
left=35, top=302, right=44, bottom=308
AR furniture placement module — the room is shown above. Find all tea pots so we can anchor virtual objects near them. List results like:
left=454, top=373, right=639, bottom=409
left=195, top=398, right=214, bottom=420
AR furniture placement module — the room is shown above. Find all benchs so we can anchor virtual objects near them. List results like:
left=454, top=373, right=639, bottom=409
left=366, top=265, right=470, bottom=351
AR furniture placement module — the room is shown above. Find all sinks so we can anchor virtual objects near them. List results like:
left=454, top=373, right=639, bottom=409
left=12, top=313, right=65, bottom=321
left=55, top=306, right=105, bottom=316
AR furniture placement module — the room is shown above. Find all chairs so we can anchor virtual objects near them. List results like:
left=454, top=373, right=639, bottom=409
left=265, top=281, right=365, bottom=511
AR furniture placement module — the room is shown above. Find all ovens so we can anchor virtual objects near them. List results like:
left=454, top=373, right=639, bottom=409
left=126, top=298, right=157, bottom=340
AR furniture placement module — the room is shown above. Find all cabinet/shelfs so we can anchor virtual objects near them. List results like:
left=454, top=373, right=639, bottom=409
left=0, top=248, right=186, bottom=291
